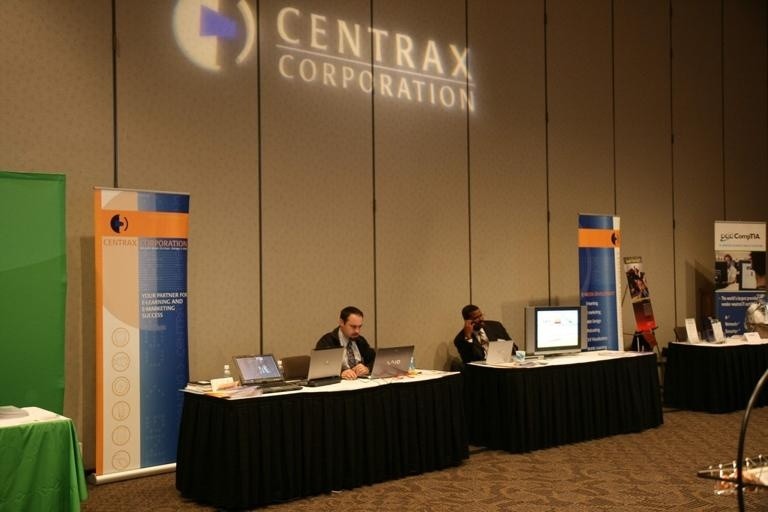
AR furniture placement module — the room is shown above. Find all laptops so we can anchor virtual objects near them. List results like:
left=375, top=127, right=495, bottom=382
left=232, top=354, right=302, bottom=393
left=298, top=346, right=345, bottom=387
left=360, top=345, right=414, bottom=379
left=471, top=340, right=514, bottom=364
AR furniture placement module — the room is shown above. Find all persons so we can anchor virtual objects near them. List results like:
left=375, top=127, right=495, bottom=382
left=453, top=304, right=518, bottom=364
left=750, top=251, right=767, bottom=290
left=722, top=253, right=736, bottom=286
left=315, top=306, right=374, bottom=381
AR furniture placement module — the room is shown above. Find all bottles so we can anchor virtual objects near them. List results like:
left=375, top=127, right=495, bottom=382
left=409, top=353, right=415, bottom=371
left=276, top=360, right=284, bottom=376
left=223, top=364, right=230, bottom=379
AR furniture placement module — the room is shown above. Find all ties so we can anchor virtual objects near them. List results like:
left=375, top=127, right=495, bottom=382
left=478, top=331, right=488, bottom=353
left=347, top=340, right=356, bottom=369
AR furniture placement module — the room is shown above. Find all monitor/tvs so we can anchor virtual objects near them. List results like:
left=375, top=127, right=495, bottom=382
left=525, top=306, right=588, bottom=355
